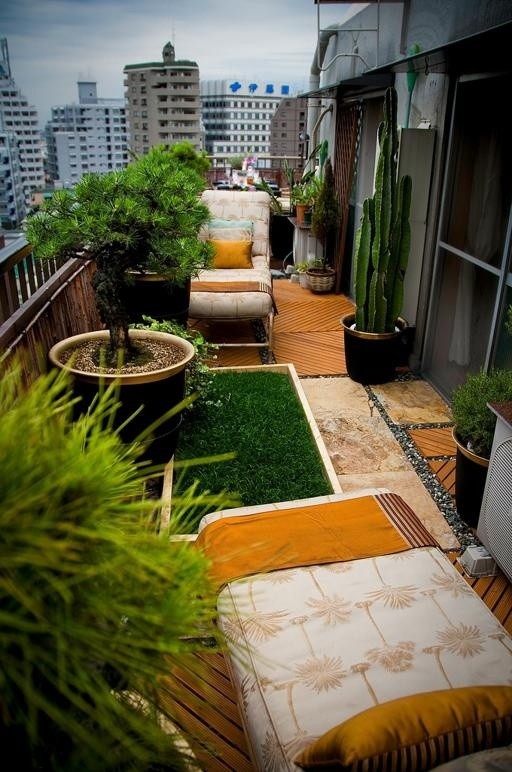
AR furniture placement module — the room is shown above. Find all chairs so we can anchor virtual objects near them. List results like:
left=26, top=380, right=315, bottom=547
left=187, top=189, right=277, bottom=366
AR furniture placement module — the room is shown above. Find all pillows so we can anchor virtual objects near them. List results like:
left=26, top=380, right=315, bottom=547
left=195, top=489, right=512, bottom=771
left=204, top=217, right=254, bottom=270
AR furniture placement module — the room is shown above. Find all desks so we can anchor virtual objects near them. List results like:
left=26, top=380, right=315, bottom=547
left=137, top=546, right=512, bottom=772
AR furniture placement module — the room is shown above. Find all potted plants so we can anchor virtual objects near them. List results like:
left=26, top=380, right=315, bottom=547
left=25, top=139, right=219, bottom=488
left=158, top=362, right=343, bottom=542
left=446, top=365, right=512, bottom=530
left=340, top=87, right=413, bottom=385
left=249, top=137, right=343, bottom=294
